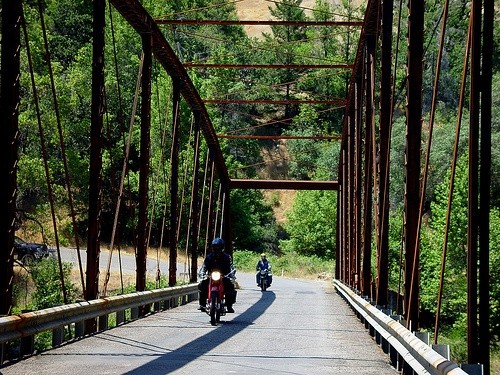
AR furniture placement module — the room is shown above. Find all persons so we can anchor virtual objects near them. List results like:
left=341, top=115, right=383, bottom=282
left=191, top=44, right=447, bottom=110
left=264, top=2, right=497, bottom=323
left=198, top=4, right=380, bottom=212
left=197, top=238, right=237, bottom=313
left=256, top=254, right=272, bottom=288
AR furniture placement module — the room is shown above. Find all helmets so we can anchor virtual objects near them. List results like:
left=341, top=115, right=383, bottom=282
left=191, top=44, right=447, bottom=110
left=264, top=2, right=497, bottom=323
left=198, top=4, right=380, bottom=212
left=212, top=238, right=224, bottom=253
left=261, top=253, right=266, bottom=259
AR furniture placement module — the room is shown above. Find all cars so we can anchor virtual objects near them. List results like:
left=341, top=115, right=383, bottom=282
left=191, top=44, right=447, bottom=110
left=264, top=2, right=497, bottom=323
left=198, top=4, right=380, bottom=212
left=13, top=235, right=47, bottom=264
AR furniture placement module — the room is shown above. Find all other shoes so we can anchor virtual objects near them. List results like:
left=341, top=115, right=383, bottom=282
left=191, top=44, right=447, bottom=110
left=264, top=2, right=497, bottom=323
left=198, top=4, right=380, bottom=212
left=228, top=308, right=234, bottom=312
left=197, top=306, right=206, bottom=310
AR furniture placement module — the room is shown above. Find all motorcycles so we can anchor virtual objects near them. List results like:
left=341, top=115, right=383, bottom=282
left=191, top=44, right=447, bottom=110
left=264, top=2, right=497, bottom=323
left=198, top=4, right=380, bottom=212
left=200, top=265, right=237, bottom=325
left=256, top=268, right=273, bottom=293
